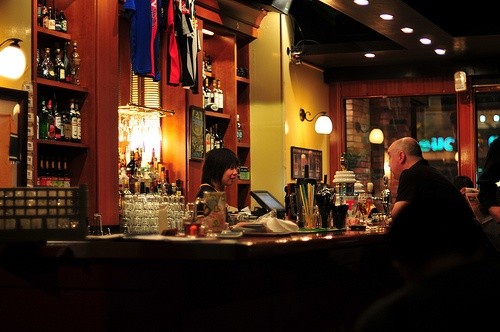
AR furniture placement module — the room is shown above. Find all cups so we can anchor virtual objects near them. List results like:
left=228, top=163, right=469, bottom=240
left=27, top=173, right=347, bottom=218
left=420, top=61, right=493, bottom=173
left=92, top=214, right=103, bottom=236
left=302, top=206, right=318, bottom=229
left=120, top=187, right=194, bottom=236
left=196, top=191, right=226, bottom=234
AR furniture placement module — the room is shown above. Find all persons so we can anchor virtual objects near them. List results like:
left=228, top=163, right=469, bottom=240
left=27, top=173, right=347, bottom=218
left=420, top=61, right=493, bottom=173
left=385, top=136, right=500, bottom=310
left=197, top=148, right=256, bottom=222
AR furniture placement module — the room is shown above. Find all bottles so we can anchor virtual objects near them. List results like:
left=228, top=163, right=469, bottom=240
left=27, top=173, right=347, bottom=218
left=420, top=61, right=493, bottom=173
left=182, top=201, right=207, bottom=237
left=36, top=160, right=71, bottom=188
left=118, top=145, right=182, bottom=197
left=37, top=0, right=67, bottom=33
left=236, top=114, right=242, bottom=143
left=230, top=211, right=249, bottom=227
left=36, top=93, right=82, bottom=143
left=36, top=38, right=81, bottom=86
left=205, top=123, right=224, bottom=153
left=202, top=50, right=223, bottom=113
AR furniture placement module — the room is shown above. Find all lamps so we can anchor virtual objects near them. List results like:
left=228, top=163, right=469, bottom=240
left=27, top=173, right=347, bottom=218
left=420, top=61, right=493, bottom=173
left=0, top=36, right=26, bottom=79
left=355, top=123, right=384, bottom=144
left=299, top=108, right=333, bottom=134
left=286, top=47, right=304, bottom=64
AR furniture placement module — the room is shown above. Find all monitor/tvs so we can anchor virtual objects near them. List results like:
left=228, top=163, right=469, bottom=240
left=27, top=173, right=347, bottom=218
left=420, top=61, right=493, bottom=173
left=249, top=190, right=288, bottom=215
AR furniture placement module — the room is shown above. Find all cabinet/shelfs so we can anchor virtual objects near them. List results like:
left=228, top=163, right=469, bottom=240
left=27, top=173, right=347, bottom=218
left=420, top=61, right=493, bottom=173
left=160, top=4, right=267, bottom=213
left=16, top=0, right=90, bottom=188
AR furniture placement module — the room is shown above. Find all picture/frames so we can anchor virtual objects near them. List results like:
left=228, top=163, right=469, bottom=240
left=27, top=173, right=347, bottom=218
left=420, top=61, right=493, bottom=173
left=290, top=146, right=324, bottom=184
